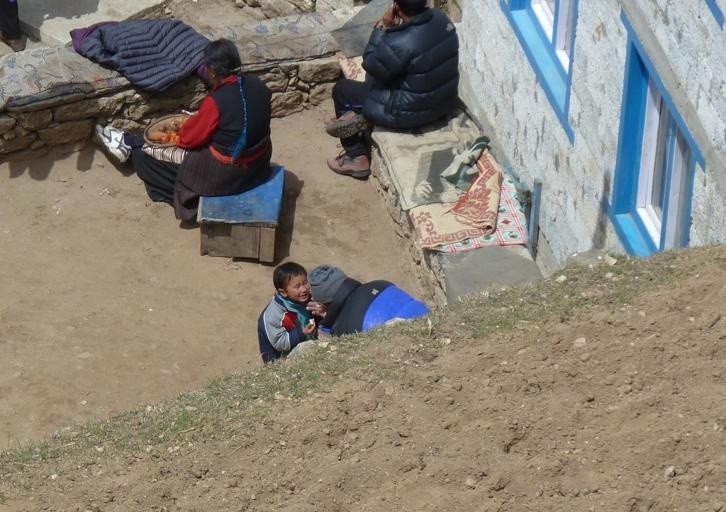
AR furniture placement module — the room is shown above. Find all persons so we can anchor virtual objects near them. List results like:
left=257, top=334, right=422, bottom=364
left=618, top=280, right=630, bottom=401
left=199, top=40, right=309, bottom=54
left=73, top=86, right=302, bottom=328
left=258, top=262, right=433, bottom=364
left=327, top=0, right=459, bottom=179
left=95, top=38, right=272, bottom=196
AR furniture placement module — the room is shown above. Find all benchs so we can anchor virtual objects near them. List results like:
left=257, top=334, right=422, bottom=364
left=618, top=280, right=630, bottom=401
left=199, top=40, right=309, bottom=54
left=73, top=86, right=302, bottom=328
left=337, top=58, right=544, bottom=314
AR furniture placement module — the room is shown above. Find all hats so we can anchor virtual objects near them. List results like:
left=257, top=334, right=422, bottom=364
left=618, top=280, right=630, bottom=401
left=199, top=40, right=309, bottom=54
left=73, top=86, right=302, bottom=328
left=307, top=264, right=350, bottom=306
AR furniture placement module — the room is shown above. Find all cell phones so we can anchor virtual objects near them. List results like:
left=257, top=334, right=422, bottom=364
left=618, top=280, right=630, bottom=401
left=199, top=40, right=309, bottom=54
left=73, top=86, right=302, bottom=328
left=393, top=3, right=395, bottom=18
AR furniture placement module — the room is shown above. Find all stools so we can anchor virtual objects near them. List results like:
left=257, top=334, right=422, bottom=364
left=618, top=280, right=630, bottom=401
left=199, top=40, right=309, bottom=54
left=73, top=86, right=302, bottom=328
left=197, top=165, right=285, bottom=264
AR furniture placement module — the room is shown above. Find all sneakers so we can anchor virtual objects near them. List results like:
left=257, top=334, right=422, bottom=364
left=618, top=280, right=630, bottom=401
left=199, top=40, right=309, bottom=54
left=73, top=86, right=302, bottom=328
left=0, top=29, right=27, bottom=52
left=326, top=111, right=368, bottom=139
left=326, top=153, right=371, bottom=179
left=94, top=123, right=132, bottom=166
